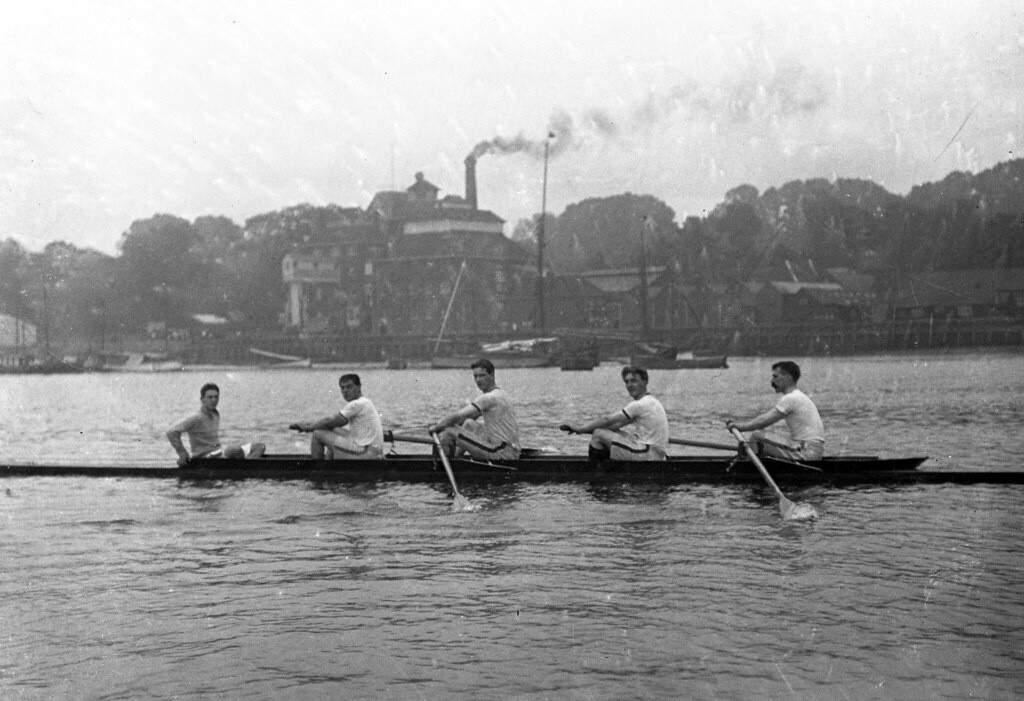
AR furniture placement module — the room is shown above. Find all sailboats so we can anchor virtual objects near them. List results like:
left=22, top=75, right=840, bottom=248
left=628, top=214, right=730, bottom=372
left=429, top=146, right=601, bottom=372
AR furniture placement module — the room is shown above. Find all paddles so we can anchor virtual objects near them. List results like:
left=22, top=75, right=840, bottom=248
left=559, top=423, right=740, bottom=452
left=728, top=417, right=799, bottom=516
left=429, top=427, right=474, bottom=513
left=289, top=422, right=434, bottom=445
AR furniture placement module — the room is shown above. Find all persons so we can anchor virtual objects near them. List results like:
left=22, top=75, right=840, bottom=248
left=564, top=366, right=669, bottom=461
left=428, top=359, right=522, bottom=461
left=728, top=361, right=825, bottom=461
left=293, top=373, right=385, bottom=459
left=166, top=384, right=265, bottom=466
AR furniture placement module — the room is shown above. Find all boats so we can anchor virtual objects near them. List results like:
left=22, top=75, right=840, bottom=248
left=101, top=358, right=182, bottom=373
left=246, top=346, right=311, bottom=370
left=0, top=450, right=1024, bottom=486
